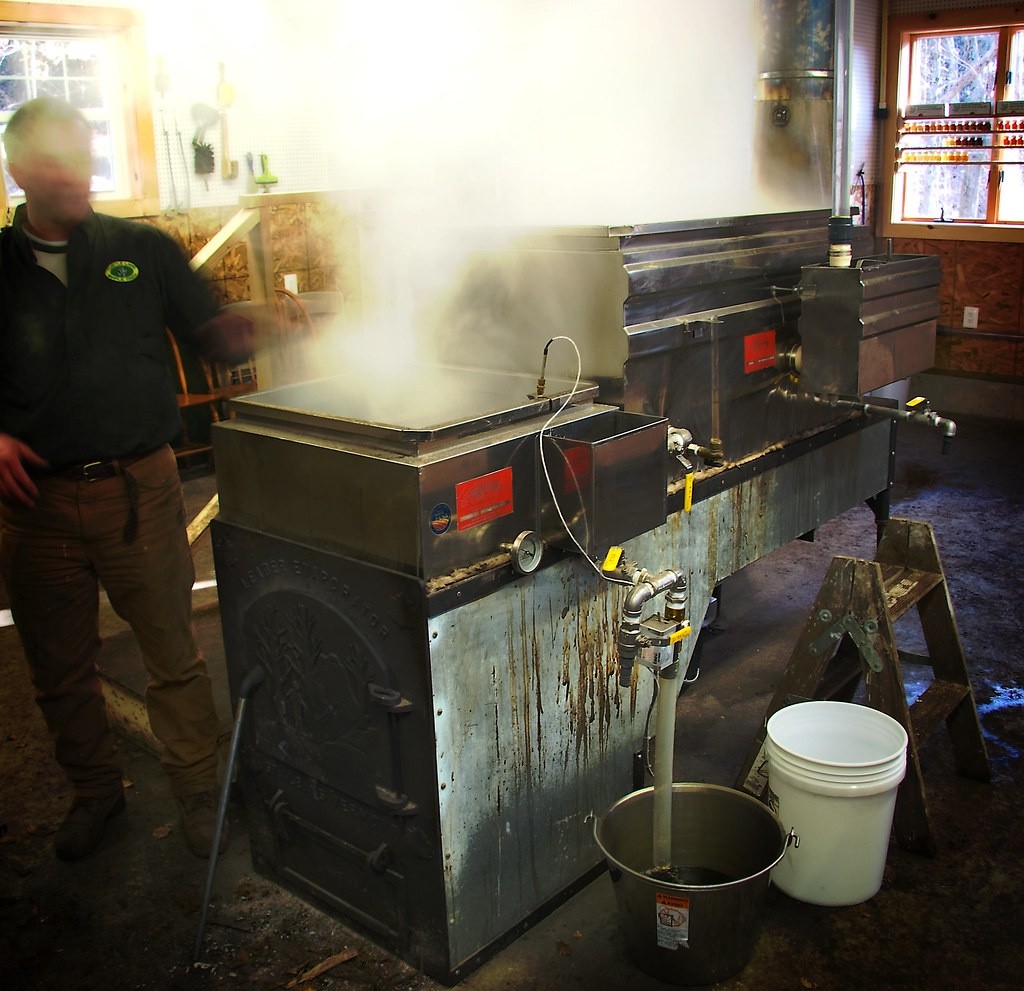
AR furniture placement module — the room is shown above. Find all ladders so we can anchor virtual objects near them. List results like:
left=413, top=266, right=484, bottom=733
left=732, top=515, right=997, bottom=865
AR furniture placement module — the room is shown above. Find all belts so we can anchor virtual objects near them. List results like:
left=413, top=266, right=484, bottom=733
left=21, top=441, right=166, bottom=544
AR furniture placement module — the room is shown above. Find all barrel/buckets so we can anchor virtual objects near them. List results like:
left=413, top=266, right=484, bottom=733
left=583, top=782, right=800, bottom=987
left=765, top=700, right=909, bottom=907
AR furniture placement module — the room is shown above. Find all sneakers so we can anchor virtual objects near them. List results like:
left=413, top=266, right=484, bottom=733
left=184, top=790, right=232, bottom=858
left=55, top=789, right=126, bottom=861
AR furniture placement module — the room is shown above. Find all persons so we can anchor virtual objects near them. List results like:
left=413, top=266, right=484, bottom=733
left=0, top=96, right=291, bottom=872
